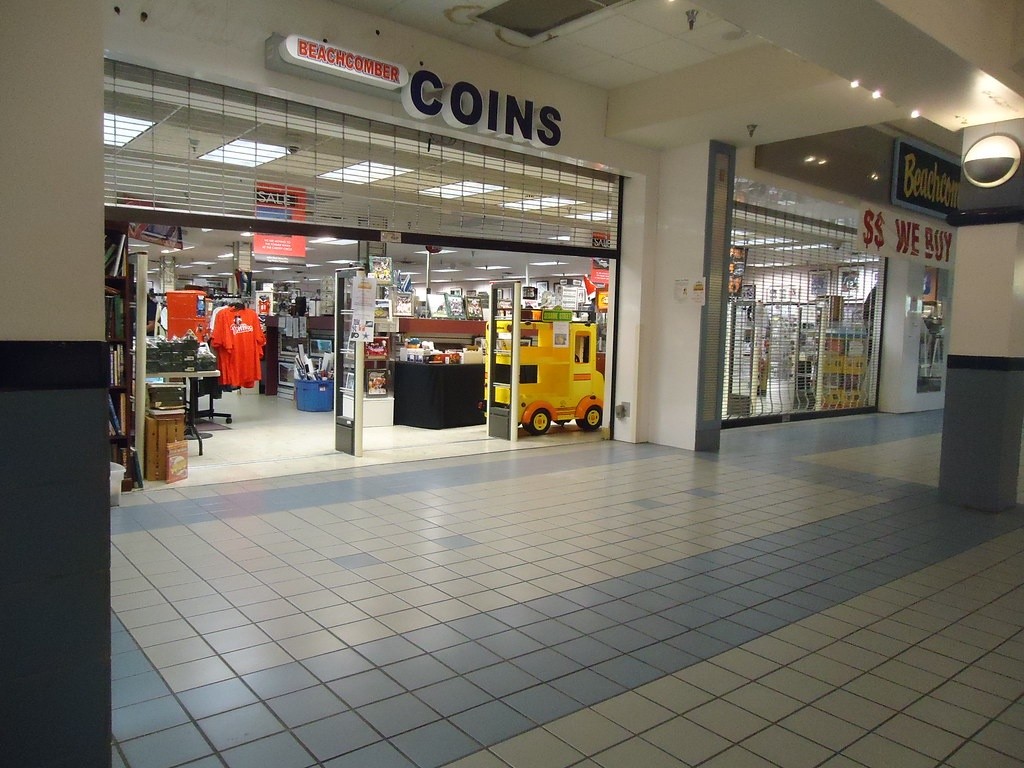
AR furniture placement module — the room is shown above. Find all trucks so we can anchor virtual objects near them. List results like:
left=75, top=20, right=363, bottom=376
left=478, top=310, right=604, bottom=436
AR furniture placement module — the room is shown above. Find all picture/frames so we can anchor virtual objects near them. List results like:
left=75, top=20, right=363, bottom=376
left=366, top=369, right=388, bottom=397
left=278, top=338, right=334, bottom=388
left=807, top=270, right=832, bottom=303
left=392, top=292, right=415, bottom=317
left=837, top=264, right=865, bottom=301
left=465, top=296, right=483, bottom=320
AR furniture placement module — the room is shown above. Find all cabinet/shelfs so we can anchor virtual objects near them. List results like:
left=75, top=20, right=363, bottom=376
left=104, top=220, right=134, bottom=492
left=364, top=269, right=394, bottom=425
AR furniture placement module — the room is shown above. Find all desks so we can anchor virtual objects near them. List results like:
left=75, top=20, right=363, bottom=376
left=146, top=370, right=221, bottom=455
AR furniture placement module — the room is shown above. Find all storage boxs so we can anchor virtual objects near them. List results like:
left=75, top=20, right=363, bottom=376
left=292, top=379, right=334, bottom=412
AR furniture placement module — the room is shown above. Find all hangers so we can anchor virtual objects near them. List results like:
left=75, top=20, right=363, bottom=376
left=210, top=294, right=246, bottom=309
